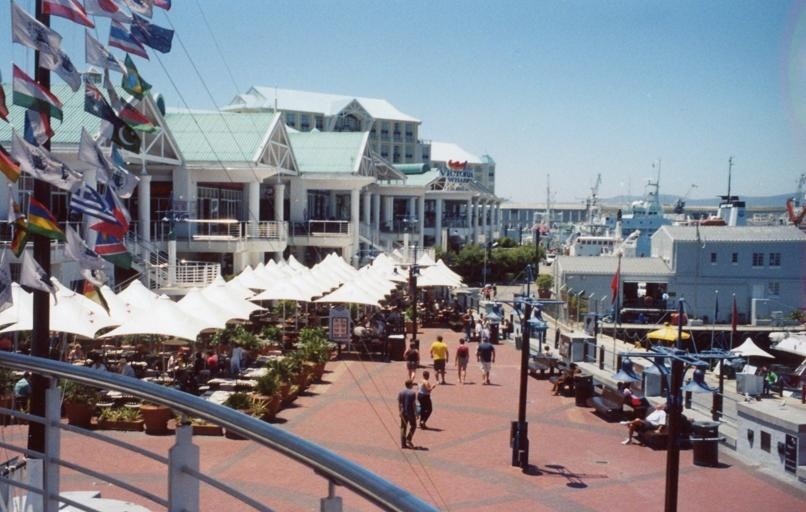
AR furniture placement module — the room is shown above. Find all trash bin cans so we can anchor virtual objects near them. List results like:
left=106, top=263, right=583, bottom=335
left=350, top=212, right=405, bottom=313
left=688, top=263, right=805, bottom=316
left=692, top=421, right=720, bottom=467
left=575, top=373, right=594, bottom=407
left=490, top=322, right=499, bottom=343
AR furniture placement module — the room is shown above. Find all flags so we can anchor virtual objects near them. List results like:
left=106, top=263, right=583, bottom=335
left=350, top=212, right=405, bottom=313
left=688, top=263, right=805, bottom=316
left=0, top=0, right=177, bottom=314
left=612, top=271, right=618, bottom=304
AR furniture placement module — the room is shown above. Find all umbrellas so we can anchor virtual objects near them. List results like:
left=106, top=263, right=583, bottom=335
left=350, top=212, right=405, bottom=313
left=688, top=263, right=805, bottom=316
left=731, top=336, right=777, bottom=366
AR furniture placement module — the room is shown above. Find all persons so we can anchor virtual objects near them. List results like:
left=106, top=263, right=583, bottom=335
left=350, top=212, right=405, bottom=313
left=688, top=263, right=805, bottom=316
left=482, top=282, right=499, bottom=300
left=15, top=345, right=254, bottom=425
left=398, top=308, right=513, bottom=449
left=543, top=345, right=552, bottom=357
left=619, top=403, right=668, bottom=446
left=550, top=363, right=582, bottom=395
left=763, top=369, right=777, bottom=395
left=617, top=382, right=650, bottom=406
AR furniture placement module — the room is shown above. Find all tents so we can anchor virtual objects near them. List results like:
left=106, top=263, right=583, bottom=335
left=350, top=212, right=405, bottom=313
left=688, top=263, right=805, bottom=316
left=0, top=249, right=462, bottom=381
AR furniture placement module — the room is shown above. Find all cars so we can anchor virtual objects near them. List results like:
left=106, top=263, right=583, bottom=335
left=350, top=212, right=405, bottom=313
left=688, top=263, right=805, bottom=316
left=543, top=253, right=556, bottom=266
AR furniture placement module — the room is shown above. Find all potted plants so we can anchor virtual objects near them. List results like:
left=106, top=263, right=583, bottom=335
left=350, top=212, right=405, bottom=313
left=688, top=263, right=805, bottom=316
left=57, top=322, right=329, bottom=439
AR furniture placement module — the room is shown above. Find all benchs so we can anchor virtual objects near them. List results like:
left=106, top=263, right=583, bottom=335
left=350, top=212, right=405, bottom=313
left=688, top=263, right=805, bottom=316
left=531, top=352, right=670, bottom=451
